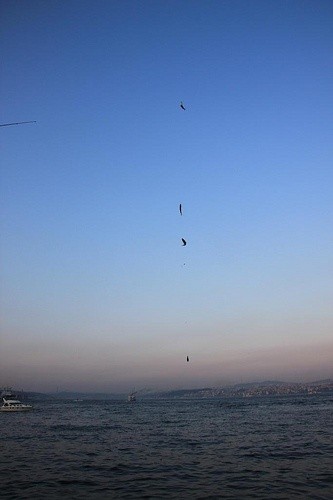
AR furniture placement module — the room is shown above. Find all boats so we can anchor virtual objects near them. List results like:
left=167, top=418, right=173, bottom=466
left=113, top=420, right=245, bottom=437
left=127, top=393, right=136, bottom=401
left=0, top=395, right=33, bottom=412
